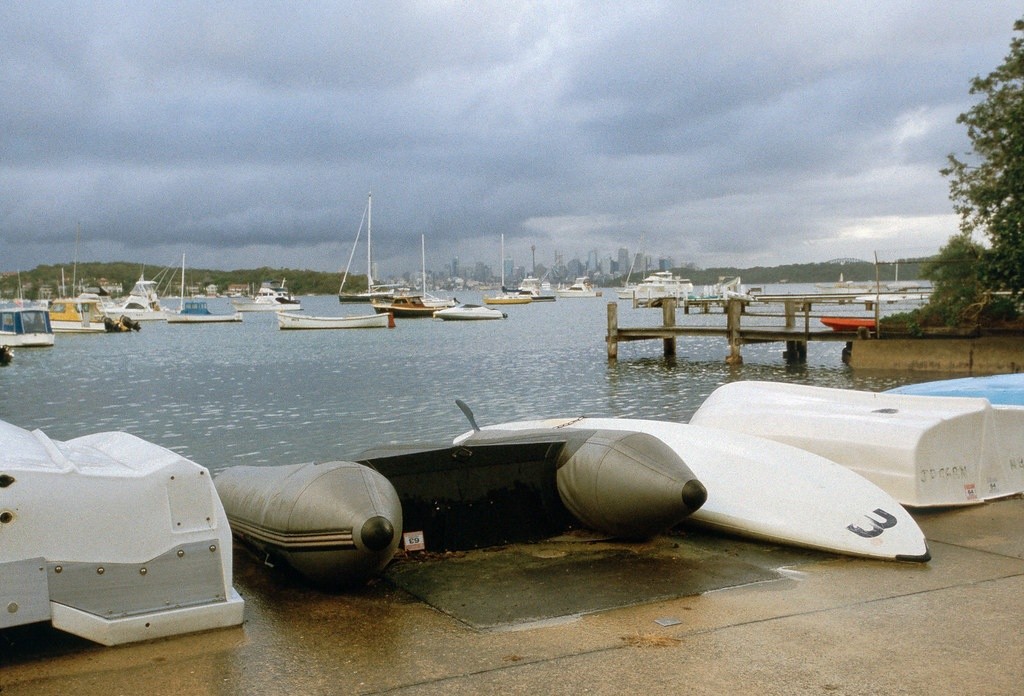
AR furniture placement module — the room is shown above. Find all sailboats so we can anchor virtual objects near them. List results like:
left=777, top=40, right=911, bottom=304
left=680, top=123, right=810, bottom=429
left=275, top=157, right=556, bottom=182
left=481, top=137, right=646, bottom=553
left=393, top=234, right=457, bottom=309
left=616, top=231, right=649, bottom=298
left=483, top=232, right=532, bottom=305
left=168, top=251, right=244, bottom=322
left=338, top=192, right=394, bottom=302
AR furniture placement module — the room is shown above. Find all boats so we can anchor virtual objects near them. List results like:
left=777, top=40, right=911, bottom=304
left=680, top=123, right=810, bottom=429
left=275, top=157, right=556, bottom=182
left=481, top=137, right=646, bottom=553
left=690, top=381, right=1024, bottom=508
left=556, top=277, right=601, bottom=298
left=821, top=318, right=877, bottom=331
left=434, top=303, right=505, bottom=321
left=215, top=396, right=708, bottom=588
left=232, top=282, right=301, bottom=313
left=0, top=420, right=245, bottom=649
left=453, top=416, right=932, bottom=564
left=373, top=288, right=445, bottom=317
left=0, top=307, right=56, bottom=347
left=100, top=282, right=179, bottom=319
left=817, top=284, right=890, bottom=293
left=46, top=299, right=142, bottom=332
left=882, top=373, right=1024, bottom=404
left=277, top=312, right=395, bottom=328
left=514, top=279, right=556, bottom=303
left=633, top=271, right=694, bottom=303
